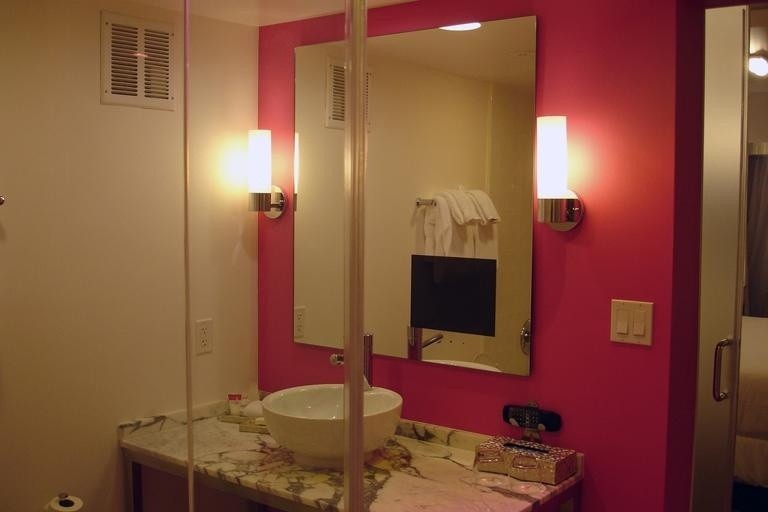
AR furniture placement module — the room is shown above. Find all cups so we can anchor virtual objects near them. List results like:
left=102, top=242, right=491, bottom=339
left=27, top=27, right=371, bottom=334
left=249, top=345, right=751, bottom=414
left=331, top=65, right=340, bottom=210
left=472, top=446, right=540, bottom=494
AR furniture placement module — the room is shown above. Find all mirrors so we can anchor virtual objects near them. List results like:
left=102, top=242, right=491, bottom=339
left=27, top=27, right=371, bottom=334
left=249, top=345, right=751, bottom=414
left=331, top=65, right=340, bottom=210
left=293, top=17, right=538, bottom=377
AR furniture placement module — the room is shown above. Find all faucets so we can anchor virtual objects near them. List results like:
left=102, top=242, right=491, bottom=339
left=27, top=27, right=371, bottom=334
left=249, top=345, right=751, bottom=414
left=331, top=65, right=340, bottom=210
left=406, top=325, right=443, bottom=361
left=330, top=332, right=372, bottom=390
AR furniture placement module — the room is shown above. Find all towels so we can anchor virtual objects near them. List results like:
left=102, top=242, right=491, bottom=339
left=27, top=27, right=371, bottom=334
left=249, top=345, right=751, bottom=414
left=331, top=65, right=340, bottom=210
left=425, top=189, right=501, bottom=268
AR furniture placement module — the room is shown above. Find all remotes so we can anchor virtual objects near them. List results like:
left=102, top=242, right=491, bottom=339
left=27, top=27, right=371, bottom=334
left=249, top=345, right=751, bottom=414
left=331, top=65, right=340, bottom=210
left=502, top=404, right=561, bottom=432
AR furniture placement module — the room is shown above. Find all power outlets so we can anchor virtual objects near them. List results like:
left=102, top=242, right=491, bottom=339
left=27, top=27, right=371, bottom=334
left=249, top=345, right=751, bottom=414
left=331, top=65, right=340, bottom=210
left=295, top=305, right=306, bottom=337
left=195, top=318, right=213, bottom=355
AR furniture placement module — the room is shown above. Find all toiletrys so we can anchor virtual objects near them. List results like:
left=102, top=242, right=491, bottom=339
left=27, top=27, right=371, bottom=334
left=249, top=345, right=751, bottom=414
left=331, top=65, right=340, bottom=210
left=227, top=393, right=242, bottom=418
left=241, top=394, right=249, bottom=407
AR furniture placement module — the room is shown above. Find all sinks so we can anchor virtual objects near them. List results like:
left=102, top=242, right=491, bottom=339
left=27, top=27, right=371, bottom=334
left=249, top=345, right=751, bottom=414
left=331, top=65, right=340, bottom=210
left=425, top=359, right=502, bottom=372
left=263, top=383, right=403, bottom=469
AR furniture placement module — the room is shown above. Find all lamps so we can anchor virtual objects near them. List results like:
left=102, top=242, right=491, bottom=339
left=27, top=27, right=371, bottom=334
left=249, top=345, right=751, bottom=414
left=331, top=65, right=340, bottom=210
left=537, top=116, right=584, bottom=232
left=247, top=130, right=286, bottom=219
